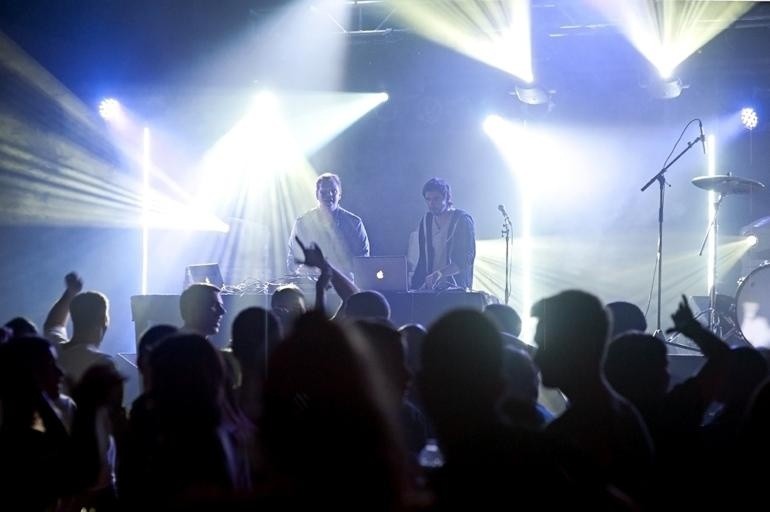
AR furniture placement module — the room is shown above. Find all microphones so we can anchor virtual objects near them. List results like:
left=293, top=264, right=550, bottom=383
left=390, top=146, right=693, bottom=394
left=698, top=123, right=706, bottom=155
left=498, top=204, right=509, bottom=221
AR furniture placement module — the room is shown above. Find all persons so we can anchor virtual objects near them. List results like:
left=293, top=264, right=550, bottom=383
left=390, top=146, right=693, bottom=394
left=411, top=176, right=476, bottom=291
left=506, top=290, right=654, bottom=511
left=293, top=236, right=392, bottom=330
left=1, top=336, right=74, bottom=511
left=415, top=308, right=637, bottom=511
left=349, top=318, right=426, bottom=457
left=177, top=285, right=225, bottom=340
left=603, top=295, right=730, bottom=512
left=232, top=307, right=283, bottom=426
left=4, top=317, right=39, bottom=339
left=122, top=334, right=256, bottom=511
left=485, top=304, right=516, bottom=336
left=43, top=272, right=132, bottom=511
left=1, top=325, right=14, bottom=344
left=500, top=332, right=555, bottom=427
left=185, top=311, right=430, bottom=510
left=496, top=346, right=548, bottom=431
left=606, top=302, right=647, bottom=341
left=113, top=323, right=177, bottom=432
left=394, top=323, right=432, bottom=413
left=271, top=267, right=333, bottom=343
left=706, top=347, right=769, bottom=511
left=287, top=172, right=370, bottom=276
left=739, top=377, right=769, bottom=512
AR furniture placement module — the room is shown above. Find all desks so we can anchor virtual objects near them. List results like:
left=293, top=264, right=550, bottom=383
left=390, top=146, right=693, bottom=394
left=132, top=292, right=493, bottom=353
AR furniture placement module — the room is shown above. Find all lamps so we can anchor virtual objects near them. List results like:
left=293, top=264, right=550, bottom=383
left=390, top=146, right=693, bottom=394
left=640, top=71, right=694, bottom=102
left=509, top=75, right=559, bottom=117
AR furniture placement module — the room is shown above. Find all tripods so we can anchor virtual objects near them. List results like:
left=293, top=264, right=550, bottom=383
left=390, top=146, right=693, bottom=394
left=665, top=221, right=746, bottom=346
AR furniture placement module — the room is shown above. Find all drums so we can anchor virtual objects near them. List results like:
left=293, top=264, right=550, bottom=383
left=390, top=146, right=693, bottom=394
left=734, top=260, right=770, bottom=352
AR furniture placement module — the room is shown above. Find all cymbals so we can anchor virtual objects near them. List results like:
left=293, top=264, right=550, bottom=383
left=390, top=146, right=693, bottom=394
left=692, top=174, right=765, bottom=192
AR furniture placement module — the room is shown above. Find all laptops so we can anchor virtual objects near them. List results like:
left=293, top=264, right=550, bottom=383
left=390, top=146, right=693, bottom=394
left=189, top=264, right=224, bottom=288
left=353, top=254, right=434, bottom=293
